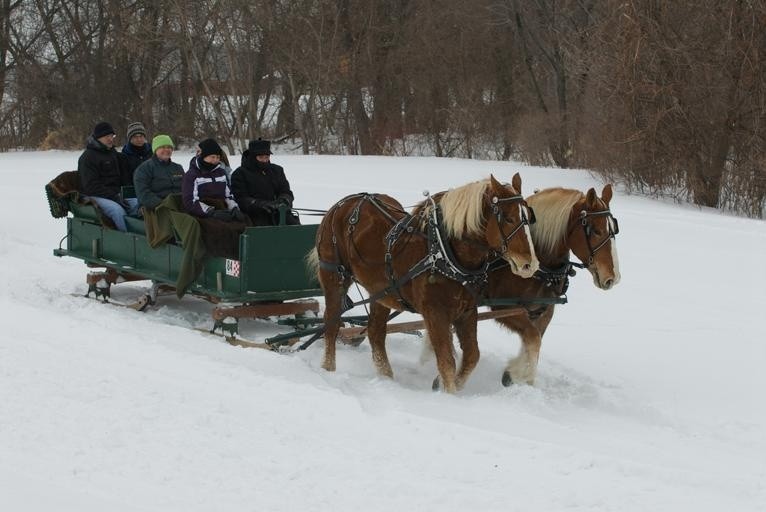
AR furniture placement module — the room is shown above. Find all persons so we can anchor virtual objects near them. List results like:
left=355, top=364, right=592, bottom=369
left=78, top=122, right=301, bottom=260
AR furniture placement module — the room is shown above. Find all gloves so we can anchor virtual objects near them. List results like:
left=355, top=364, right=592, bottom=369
left=253, top=196, right=290, bottom=215
left=231, top=207, right=246, bottom=223
left=210, top=208, right=232, bottom=223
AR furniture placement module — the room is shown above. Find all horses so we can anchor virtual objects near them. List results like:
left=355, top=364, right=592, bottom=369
left=410, top=183, right=621, bottom=388
left=306, top=171, right=540, bottom=398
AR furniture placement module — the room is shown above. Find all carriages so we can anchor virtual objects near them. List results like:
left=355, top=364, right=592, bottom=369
left=54, top=171, right=621, bottom=400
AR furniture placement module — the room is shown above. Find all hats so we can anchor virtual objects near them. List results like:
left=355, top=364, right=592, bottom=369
left=94, top=121, right=115, bottom=138
left=198, top=137, right=222, bottom=157
left=126, top=121, right=147, bottom=142
left=248, top=136, right=274, bottom=158
left=151, top=134, right=176, bottom=155
